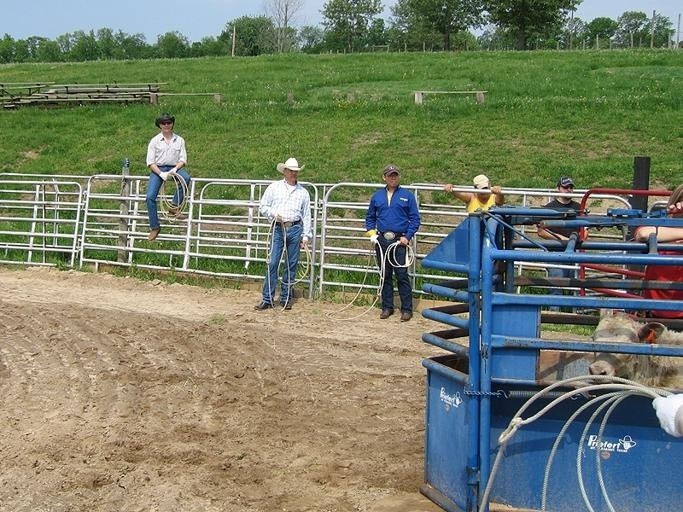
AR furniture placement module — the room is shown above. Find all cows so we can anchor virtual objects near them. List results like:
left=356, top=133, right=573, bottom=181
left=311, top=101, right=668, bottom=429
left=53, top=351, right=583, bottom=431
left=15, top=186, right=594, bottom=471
left=585, top=306, right=683, bottom=393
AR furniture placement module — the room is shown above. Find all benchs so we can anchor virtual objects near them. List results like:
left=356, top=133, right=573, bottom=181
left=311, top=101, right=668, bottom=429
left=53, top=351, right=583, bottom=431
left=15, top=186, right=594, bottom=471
left=0, top=75, right=223, bottom=110
left=412, top=85, right=488, bottom=109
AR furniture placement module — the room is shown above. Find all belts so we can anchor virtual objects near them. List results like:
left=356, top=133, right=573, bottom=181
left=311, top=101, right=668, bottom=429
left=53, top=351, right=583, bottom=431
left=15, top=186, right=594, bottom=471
left=381, top=230, right=405, bottom=241
left=277, top=220, right=300, bottom=228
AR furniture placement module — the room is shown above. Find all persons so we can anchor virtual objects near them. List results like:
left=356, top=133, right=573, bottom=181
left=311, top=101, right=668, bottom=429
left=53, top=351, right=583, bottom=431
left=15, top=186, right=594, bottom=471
left=362, top=165, right=422, bottom=322
left=443, top=173, right=503, bottom=216
left=143, top=112, right=190, bottom=244
left=535, top=176, right=589, bottom=311
left=253, top=157, right=312, bottom=312
left=648, top=391, right=683, bottom=439
left=632, top=182, right=683, bottom=333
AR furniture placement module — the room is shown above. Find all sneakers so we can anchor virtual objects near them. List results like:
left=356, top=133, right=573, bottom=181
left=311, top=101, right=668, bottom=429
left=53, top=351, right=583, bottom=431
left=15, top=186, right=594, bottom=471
left=379, top=308, right=393, bottom=319
left=148, top=228, right=161, bottom=241
left=401, top=312, right=412, bottom=322
left=170, top=204, right=182, bottom=218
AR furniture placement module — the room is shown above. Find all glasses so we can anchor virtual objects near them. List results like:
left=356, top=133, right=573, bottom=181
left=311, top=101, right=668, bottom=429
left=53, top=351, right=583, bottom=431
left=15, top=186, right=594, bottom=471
left=161, top=121, right=172, bottom=125
left=561, top=185, right=573, bottom=189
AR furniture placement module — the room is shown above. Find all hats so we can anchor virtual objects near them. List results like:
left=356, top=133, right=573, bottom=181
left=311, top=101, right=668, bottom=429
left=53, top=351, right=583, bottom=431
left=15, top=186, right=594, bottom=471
left=276, top=157, right=305, bottom=175
left=155, top=113, right=175, bottom=129
left=474, top=174, right=489, bottom=189
left=558, top=176, right=576, bottom=188
left=383, top=165, right=400, bottom=177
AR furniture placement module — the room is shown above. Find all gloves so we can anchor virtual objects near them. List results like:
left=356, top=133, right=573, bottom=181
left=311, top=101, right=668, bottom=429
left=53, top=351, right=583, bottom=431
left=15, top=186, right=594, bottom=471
left=652, top=393, right=683, bottom=439
left=159, top=167, right=178, bottom=181
left=371, top=234, right=379, bottom=243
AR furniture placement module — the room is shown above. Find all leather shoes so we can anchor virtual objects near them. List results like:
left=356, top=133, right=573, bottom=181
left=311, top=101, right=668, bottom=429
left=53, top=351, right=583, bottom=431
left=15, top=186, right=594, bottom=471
left=283, top=302, right=291, bottom=309
left=255, top=302, right=274, bottom=310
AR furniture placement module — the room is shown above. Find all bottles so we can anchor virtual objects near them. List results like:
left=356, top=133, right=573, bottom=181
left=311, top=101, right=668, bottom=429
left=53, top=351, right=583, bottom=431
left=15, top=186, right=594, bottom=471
left=121, top=157, right=130, bottom=176
